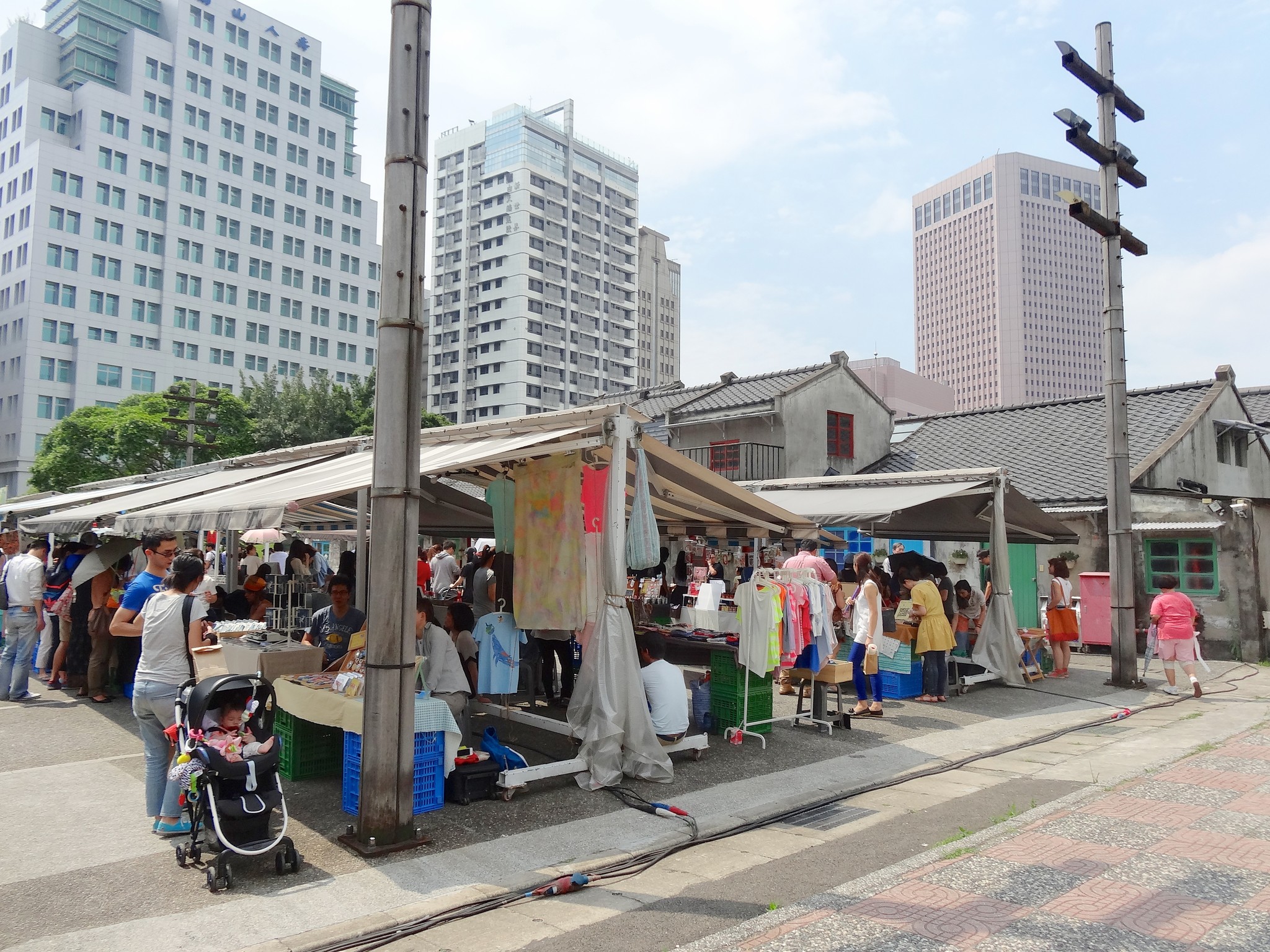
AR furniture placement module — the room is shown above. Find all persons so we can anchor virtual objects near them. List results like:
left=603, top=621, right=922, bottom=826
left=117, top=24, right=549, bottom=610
left=845, top=552, right=883, bottom=718
left=637, top=633, right=690, bottom=746
left=1045, top=555, right=1074, bottom=678
left=1150, top=573, right=1202, bottom=698
left=781, top=540, right=837, bottom=696
left=900, top=568, right=959, bottom=701
left=212, top=695, right=274, bottom=762
left=0, top=527, right=574, bottom=715
left=632, top=542, right=994, bottom=633
left=131, top=554, right=216, bottom=836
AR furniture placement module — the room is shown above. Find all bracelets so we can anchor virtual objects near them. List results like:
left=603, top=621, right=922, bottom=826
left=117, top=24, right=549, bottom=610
left=913, top=610, right=916, bottom=615
left=1046, top=607, right=1052, bottom=610
left=866, top=634, right=873, bottom=639
left=976, top=624, right=982, bottom=628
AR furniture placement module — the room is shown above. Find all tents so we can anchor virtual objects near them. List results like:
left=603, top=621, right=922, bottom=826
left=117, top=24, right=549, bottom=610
left=0, top=404, right=1082, bottom=532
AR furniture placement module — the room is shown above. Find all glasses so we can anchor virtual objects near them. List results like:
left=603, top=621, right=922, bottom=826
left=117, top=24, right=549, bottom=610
left=329, top=590, right=349, bottom=596
left=453, top=549, right=455, bottom=552
left=150, top=547, right=181, bottom=557
left=710, top=557, right=715, bottom=559
left=896, top=548, right=905, bottom=552
left=978, top=556, right=988, bottom=562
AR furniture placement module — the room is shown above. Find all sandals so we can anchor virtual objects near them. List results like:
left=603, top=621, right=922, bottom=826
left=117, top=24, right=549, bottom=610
left=916, top=695, right=946, bottom=702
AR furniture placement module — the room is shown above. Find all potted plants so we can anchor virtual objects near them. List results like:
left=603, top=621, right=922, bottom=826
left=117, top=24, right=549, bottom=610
left=1057, top=550, right=1079, bottom=568
left=952, top=549, right=968, bottom=565
left=872, top=548, right=888, bottom=562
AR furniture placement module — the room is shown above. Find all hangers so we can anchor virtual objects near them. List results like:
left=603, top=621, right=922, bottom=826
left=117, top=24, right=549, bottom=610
left=739, top=567, right=829, bottom=593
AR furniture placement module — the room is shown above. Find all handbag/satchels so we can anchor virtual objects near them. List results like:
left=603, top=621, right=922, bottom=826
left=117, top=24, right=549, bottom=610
left=849, top=603, right=896, bottom=632
left=0, top=581, right=9, bottom=611
left=50, top=581, right=74, bottom=623
left=88, top=606, right=119, bottom=640
left=1046, top=608, right=1079, bottom=642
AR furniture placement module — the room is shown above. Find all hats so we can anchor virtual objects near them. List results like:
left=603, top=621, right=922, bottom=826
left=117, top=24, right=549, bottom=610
left=80, top=532, right=103, bottom=546
left=842, top=552, right=855, bottom=564
left=244, top=576, right=266, bottom=591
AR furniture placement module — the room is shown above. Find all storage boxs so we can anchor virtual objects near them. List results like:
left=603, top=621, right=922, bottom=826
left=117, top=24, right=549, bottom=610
left=880, top=658, right=924, bottom=700
left=272, top=705, right=344, bottom=782
left=342, top=727, right=445, bottom=815
left=787, top=657, right=853, bottom=683
left=708, top=647, right=774, bottom=737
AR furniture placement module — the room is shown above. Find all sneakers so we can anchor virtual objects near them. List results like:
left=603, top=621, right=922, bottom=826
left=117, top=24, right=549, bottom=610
left=1044, top=668, right=1069, bottom=679
left=1164, top=686, right=1178, bottom=695
left=39, top=675, right=50, bottom=681
left=46, top=681, right=79, bottom=689
left=0, top=691, right=41, bottom=701
left=152, top=819, right=203, bottom=833
left=1190, top=676, right=1202, bottom=698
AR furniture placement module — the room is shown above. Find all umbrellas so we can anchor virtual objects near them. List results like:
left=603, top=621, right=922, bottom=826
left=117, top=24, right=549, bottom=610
left=1143, top=623, right=1157, bottom=677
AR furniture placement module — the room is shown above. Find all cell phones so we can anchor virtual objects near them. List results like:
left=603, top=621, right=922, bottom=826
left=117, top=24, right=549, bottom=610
left=455, top=747, right=473, bottom=758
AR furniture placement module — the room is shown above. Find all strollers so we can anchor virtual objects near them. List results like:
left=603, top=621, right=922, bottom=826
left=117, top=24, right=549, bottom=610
left=170, top=672, right=298, bottom=892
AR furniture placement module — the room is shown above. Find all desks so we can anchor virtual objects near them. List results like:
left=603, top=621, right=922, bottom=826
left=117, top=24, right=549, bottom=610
left=1018, top=630, right=1049, bottom=684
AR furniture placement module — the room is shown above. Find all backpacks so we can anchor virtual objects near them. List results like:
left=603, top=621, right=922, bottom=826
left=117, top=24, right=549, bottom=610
left=44, top=556, right=86, bottom=621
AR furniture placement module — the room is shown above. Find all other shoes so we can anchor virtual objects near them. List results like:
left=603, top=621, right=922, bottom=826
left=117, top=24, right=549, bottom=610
left=802, top=686, right=811, bottom=698
left=778, top=684, right=794, bottom=694
left=774, top=680, right=798, bottom=685
left=517, top=683, right=526, bottom=691
left=77, top=693, right=88, bottom=696
left=92, top=695, right=116, bottom=703
left=845, top=708, right=883, bottom=717
left=827, top=687, right=847, bottom=694
left=546, top=698, right=569, bottom=706
left=534, top=690, right=546, bottom=698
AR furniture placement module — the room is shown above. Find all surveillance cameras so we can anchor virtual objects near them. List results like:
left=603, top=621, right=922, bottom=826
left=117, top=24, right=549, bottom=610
left=1229, top=503, right=1248, bottom=512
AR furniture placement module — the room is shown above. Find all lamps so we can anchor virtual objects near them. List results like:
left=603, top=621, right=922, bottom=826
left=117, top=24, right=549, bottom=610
left=1207, top=499, right=1225, bottom=516
